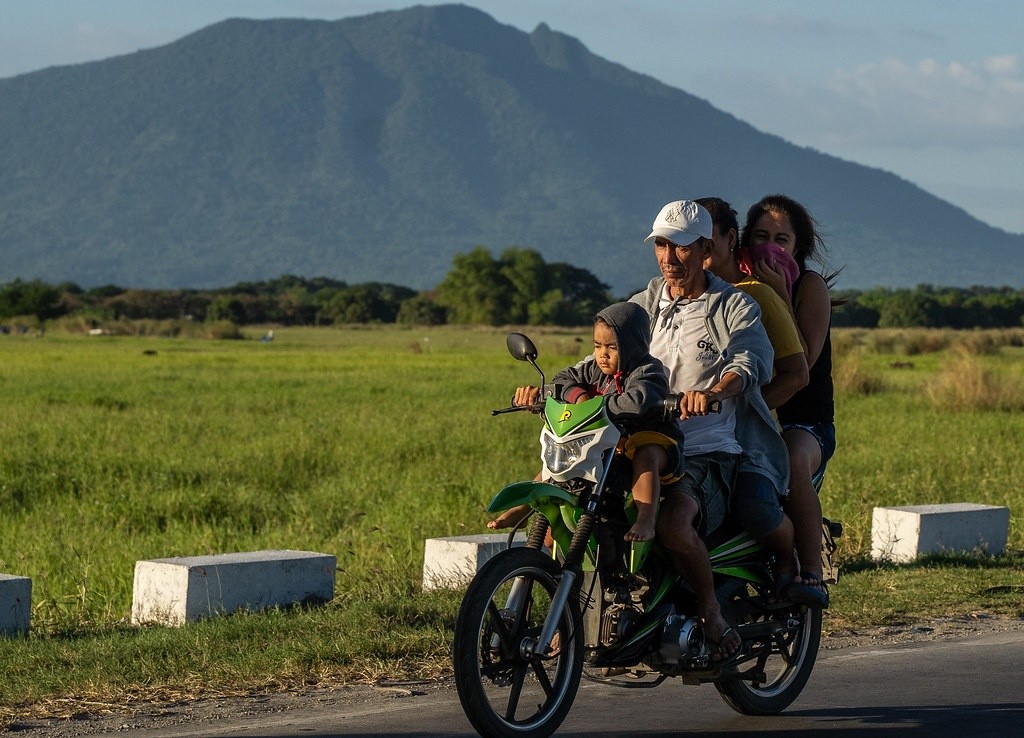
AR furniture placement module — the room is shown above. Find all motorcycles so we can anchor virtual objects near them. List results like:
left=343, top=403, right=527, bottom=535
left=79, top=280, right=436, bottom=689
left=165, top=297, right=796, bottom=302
left=450, top=331, right=846, bottom=738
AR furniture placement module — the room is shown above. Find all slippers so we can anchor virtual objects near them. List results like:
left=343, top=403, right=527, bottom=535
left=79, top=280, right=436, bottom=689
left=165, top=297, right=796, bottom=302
left=765, top=573, right=797, bottom=612
left=706, top=626, right=744, bottom=669
left=786, top=571, right=830, bottom=609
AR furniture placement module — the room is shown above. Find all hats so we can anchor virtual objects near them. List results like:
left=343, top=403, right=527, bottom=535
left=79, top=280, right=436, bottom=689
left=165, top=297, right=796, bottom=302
left=643, top=200, right=713, bottom=247
left=738, top=238, right=801, bottom=307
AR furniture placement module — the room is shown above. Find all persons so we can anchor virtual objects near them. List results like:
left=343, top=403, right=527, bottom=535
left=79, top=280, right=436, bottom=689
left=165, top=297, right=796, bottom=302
left=738, top=192, right=837, bottom=609
left=486, top=302, right=686, bottom=543
left=515, top=199, right=792, bottom=664
left=692, top=197, right=820, bottom=585
